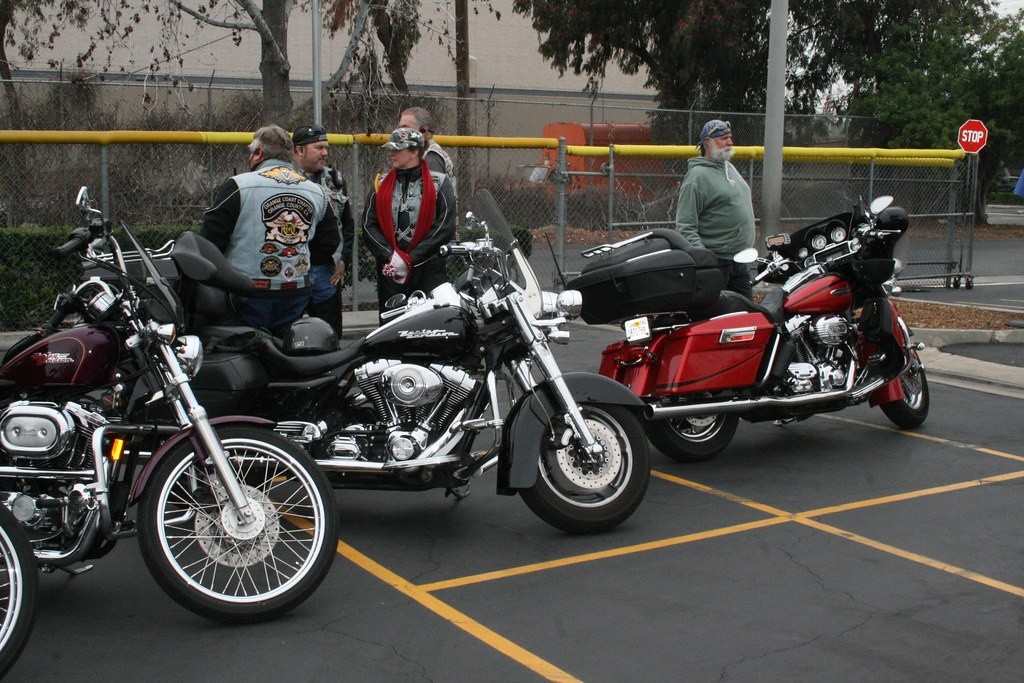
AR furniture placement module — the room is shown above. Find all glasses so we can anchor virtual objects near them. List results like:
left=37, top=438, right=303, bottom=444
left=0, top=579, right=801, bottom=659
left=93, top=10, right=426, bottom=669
left=708, top=121, right=731, bottom=137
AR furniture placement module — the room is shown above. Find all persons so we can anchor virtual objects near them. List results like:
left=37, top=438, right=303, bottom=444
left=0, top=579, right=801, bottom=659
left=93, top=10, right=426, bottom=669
left=289, top=121, right=353, bottom=339
left=198, top=123, right=340, bottom=342
left=676, top=119, right=754, bottom=300
left=397, top=104, right=459, bottom=290
left=359, top=126, right=455, bottom=329
left=993, top=158, right=1010, bottom=185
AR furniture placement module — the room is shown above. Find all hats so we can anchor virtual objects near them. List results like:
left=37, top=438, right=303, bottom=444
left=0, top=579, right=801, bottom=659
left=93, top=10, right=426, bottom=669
left=292, top=125, right=327, bottom=145
left=381, top=127, right=426, bottom=150
left=699, top=120, right=731, bottom=143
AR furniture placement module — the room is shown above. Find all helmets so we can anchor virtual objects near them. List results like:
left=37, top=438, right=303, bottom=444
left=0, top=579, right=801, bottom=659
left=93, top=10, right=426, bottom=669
left=282, top=317, right=338, bottom=354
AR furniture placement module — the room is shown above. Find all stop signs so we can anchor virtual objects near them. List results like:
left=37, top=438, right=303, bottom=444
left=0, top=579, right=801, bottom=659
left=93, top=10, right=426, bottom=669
left=957, top=119, right=988, bottom=153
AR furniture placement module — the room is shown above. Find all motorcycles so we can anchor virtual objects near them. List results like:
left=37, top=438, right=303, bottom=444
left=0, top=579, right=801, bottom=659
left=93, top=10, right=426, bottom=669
left=1, top=186, right=340, bottom=624
left=564, top=194, right=931, bottom=464
left=80, top=187, right=656, bottom=534
